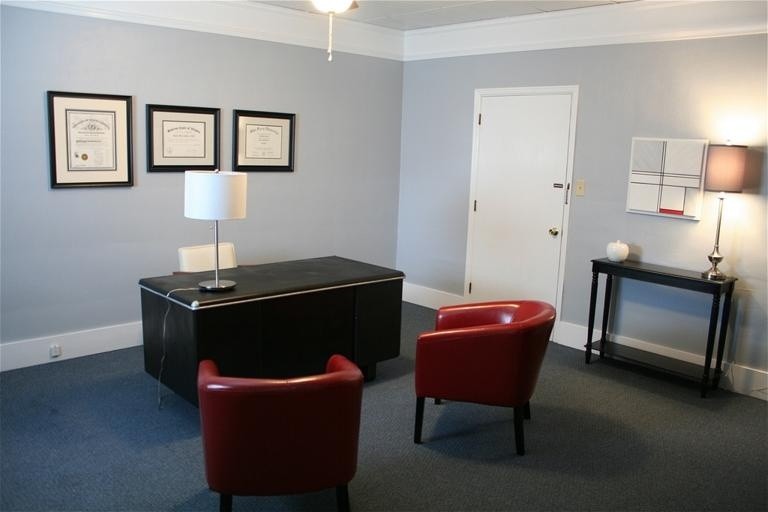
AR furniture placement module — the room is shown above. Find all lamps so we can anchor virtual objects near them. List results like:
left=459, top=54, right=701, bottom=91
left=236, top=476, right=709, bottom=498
left=700, top=142, right=748, bottom=280
left=183, top=167, right=248, bottom=292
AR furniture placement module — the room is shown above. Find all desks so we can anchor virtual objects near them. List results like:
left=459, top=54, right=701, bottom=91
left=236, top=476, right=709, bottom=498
left=138, top=255, right=406, bottom=407
left=584, top=257, right=739, bottom=398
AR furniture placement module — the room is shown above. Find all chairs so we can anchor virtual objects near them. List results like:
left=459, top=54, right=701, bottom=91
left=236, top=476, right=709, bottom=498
left=413, top=299, right=558, bottom=456
left=197, top=355, right=364, bottom=512
left=177, top=241, right=236, bottom=274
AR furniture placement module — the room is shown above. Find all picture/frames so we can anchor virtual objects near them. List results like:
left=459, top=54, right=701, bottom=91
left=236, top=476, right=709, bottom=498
left=624, top=137, right=710, bottom=222
left=46, top=89, right=298, bottom=190
